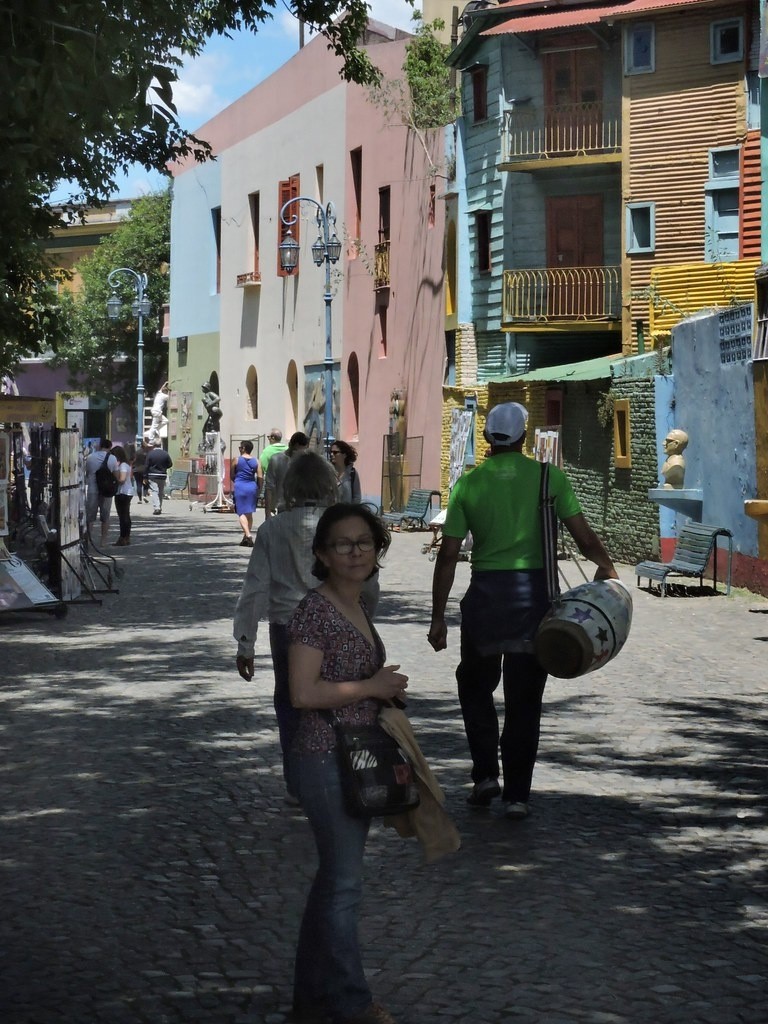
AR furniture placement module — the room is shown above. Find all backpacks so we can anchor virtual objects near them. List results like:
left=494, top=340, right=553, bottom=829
left=95, top=451, right=119, bottom=498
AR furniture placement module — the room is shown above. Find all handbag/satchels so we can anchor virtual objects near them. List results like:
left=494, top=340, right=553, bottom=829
left=334, top=722, right=421, bottom=819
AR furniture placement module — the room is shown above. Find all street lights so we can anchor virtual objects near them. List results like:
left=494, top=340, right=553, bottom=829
left=104, top=266, right=152, bottom=456
left=278, top=197, right=345, bottom=467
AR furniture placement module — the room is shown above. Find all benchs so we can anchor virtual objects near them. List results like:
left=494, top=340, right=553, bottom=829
left=634, top=522, right=734, bottom=598
left=381, top=490, right=442, bottom=533
left=14, top=494, right=54, bottom=548
left=164, top=470, right=191, bottom=500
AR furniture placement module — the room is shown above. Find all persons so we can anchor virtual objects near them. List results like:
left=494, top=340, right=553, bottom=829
left=201, top=383, right=222, bottom=444
left=428, top=401, right=620, bottom=820
left=661, top=430, right=688, bottom=489
left=28, top=381, right=170, bottom=546
left=231, top=428, right=360, bottom=547
left=234, top=449, right=380, bottom=805
left=277, top=503, right=410, bottom=1024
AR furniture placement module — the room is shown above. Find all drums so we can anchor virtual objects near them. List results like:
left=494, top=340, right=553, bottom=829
left=539, top=579, right=635, bottom=679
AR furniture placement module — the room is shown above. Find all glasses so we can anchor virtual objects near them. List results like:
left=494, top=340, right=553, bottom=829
left=267, top=436, right=272, bottom=440
left=329, top=450, right=340, bottom=455
left=239, top=447, right=243, bottom=450
left=327, top=536, right=378, bottom=556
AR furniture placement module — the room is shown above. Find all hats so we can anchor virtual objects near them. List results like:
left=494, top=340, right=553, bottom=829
left=484, top=400, right=529, bottom=445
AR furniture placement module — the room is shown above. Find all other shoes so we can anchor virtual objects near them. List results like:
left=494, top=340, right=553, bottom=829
left=240, top=534, right=254, bottom=547
left=138, top=501, right=142, bottom=504
left=153, top=509, right=161, bottom=514
left=144, top=496, right=149, bottom=503
left=333, top=998, right=396, bottom=1024
left=466, top=778, right=501, bottom=806
left=112, top=536, right=131, bottom=546
left=504, top=799, right=533, bottom=818
